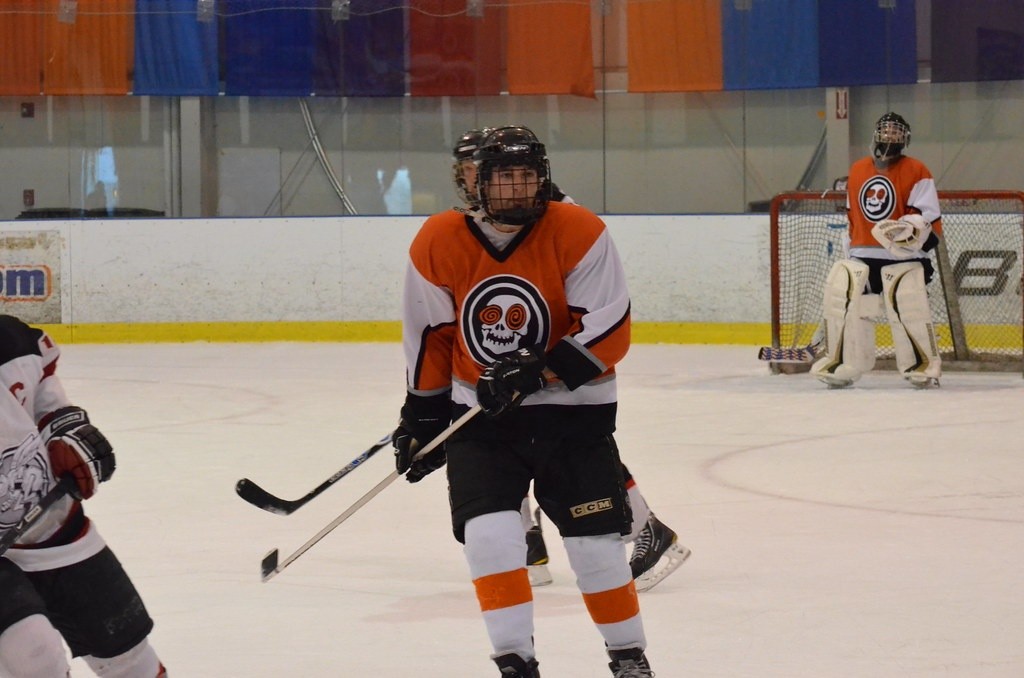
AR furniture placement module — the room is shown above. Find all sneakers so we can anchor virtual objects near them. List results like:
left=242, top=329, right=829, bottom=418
left=605, top=640, right=656, bottom=678
left=489, top=636, right=540, bottom=678
left=526, top=507, right=553, bottom=587
left=629, top=512, right=691, bottom=592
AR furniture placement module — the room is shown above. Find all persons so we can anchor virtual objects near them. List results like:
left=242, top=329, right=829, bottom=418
left=391, top=123, right=681, bottom=678
left=805, top=110, right=947, bottom=390
left=0, top=314, right=169, bottom=677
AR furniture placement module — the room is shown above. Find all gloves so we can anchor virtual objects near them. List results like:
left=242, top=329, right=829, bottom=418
left=392, top=391, right=454, bottom=483
left=38, top=406, right=116, bottom=500
left=476, top=343, right=547, bottom=422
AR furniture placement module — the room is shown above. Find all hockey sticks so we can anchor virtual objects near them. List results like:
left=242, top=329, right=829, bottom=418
left=235, top=429, right=395, bottom=518
left=760, top=326, right=829, bottom=364
left=1, top=480, right=66, bottom=556
left=262, top=398, right=483, bottom=582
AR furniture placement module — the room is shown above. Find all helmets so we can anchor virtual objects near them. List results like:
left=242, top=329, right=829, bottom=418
left=449, top=124, right=553, bottom=226
left=874, top=112, right=911, bottom=150
